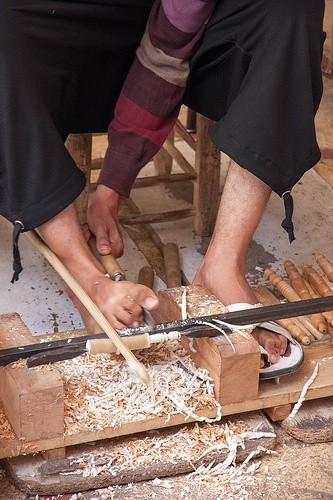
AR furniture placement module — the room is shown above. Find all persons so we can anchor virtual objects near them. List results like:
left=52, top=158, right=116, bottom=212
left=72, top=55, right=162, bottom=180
left=0, top=1, right=326, bottom=382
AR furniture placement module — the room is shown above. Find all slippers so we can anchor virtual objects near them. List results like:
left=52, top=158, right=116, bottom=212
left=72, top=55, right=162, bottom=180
left=225, top=303, right=305, bottom=380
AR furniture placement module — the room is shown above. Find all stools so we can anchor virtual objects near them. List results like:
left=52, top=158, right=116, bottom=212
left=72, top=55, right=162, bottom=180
left=64, top=111, right=222, bottom=237
